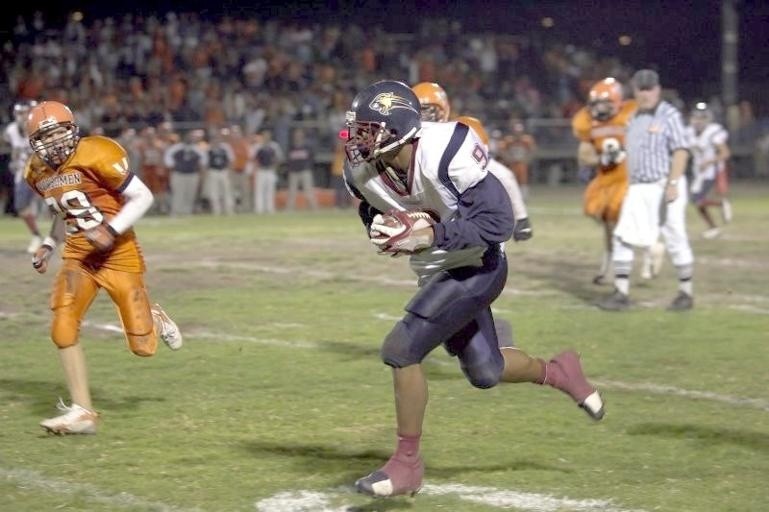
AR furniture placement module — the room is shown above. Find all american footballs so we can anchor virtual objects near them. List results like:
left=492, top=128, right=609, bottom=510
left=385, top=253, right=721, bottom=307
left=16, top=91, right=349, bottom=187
left=372, top=210, right=436, bottom=255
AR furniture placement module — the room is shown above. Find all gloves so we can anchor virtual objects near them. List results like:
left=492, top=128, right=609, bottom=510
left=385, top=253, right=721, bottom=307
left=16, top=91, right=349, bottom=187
left=514, top=218, right=532, bottom=240
left=370, top=208, right=435, bottom=260
left=32, top=245, right=53, bottom=273
left=85, top=222, right=120, bottom=253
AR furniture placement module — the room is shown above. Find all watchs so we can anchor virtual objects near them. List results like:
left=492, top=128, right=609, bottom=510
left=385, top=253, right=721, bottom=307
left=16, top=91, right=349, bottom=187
left=666, top=178, right=681, bottom=185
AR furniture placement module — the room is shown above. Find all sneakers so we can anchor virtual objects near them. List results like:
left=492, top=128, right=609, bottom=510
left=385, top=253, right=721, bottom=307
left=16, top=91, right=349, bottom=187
left=667, top=290, right=694, bottom=311
left=150, top=302, right=183, bottom=352
left=550, top=349, right=606, bottom=422
left=594, top=288, right=631, bottom=311
left=39, top=396, right=100, bottom=436
left=353, top=457, right=425, bottom=501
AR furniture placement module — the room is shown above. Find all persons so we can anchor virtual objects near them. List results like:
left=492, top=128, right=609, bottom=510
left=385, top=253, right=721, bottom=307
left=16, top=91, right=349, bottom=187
left=409, top=81, right=535, bottom=241
left=685, top=103, right=734, bottom=239
left=4, top=100, right=66, bottom=257
left=5, top=13, right=764, bottom=212
left=21, top=100, right=185, bottom=434
left=340, top=79, right=605, bottom=498
left=573, top=77, right=664, bottom=278
left=593, top=68, right=696, bottom=311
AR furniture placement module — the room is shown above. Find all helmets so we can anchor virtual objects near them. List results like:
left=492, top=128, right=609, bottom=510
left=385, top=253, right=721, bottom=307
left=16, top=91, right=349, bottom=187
left=411, top=82, right=451, bottom=125
left=27, top=100, right=82, bottom=171
left=344, top=79, right=423, bottom=170
left=585, top=78, right=624, bottom=123
left=13, top=97, right=38, bottom=131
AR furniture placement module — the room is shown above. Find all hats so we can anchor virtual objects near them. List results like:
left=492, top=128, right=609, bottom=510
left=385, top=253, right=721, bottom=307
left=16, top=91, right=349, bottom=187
left=633, top=68, right=660, bottom=91
